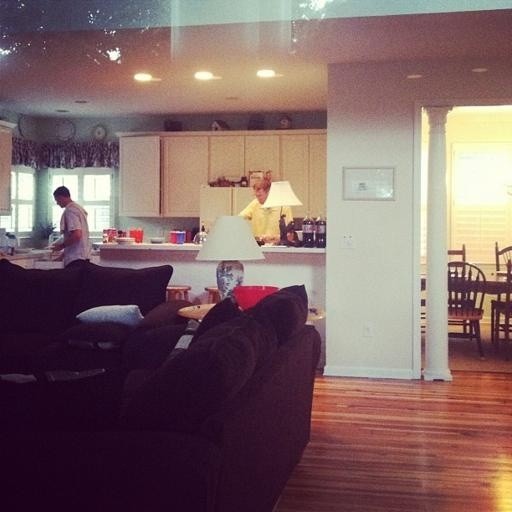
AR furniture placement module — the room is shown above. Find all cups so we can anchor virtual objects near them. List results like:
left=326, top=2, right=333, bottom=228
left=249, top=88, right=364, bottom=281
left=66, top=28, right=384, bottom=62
left=170, top=231, right=176, bottom=243
left=176, top=231, right=186, bottom=244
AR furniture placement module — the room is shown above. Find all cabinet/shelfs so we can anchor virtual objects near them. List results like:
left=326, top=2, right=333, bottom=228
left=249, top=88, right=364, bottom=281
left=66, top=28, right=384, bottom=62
left=0, top=125, right=18, bottom=215
left=280, top=135, right=327, bottom=216
left=209, top=136, right=278, bottom=216
left=120, top=136, right=209, bottom=217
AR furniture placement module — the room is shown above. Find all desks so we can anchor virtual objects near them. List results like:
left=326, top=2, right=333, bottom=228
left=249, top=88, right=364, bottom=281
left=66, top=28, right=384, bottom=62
left=176, top=301, right=326, bottom=322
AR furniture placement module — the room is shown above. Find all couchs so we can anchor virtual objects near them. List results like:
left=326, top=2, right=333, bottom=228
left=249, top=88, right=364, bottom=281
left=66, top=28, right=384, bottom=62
left=0, top=256, right=322, bottom=512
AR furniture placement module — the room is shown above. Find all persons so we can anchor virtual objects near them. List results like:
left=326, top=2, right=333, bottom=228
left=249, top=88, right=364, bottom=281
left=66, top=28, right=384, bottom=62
left=48, top=184, right=92, bottom=268
left=237, top=179, right=294, bottom=245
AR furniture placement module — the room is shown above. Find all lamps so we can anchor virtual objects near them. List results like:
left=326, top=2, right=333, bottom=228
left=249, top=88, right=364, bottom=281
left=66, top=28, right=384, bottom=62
left=258, top=180, right=303, bottom=247
left=195, top=217, right=267, bottom=307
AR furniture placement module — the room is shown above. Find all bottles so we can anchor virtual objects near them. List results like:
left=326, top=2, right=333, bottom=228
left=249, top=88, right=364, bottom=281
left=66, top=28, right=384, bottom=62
left=303, top=213, right=314, bottom=248
left=129, top=224, right=137, bottom=238
left=137, top=225, right=144, bottom=242
left=315, top=213, right=326, bottom=248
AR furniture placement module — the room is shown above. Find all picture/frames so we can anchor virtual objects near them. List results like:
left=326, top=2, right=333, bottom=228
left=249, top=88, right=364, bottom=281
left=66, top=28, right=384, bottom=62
left=342, top=166, right=397, bottom=201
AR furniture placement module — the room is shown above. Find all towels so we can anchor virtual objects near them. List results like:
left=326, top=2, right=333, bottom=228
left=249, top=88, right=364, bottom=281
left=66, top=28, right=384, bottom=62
left=59, top=202, right=88, bottom=230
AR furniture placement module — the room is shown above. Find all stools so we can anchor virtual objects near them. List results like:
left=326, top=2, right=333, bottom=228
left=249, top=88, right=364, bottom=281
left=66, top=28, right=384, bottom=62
left=204, top=285, right=220, bottom=304
left=165, top=285, right=190, bottom=306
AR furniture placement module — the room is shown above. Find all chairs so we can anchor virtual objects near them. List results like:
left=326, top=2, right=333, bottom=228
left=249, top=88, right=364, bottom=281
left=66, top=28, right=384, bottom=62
left=418, top=240, right=512, bottom=361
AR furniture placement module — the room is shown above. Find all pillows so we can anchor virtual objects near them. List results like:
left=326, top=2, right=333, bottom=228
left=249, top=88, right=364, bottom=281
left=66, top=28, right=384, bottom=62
left=73, top=304, right=142, bottom=328
